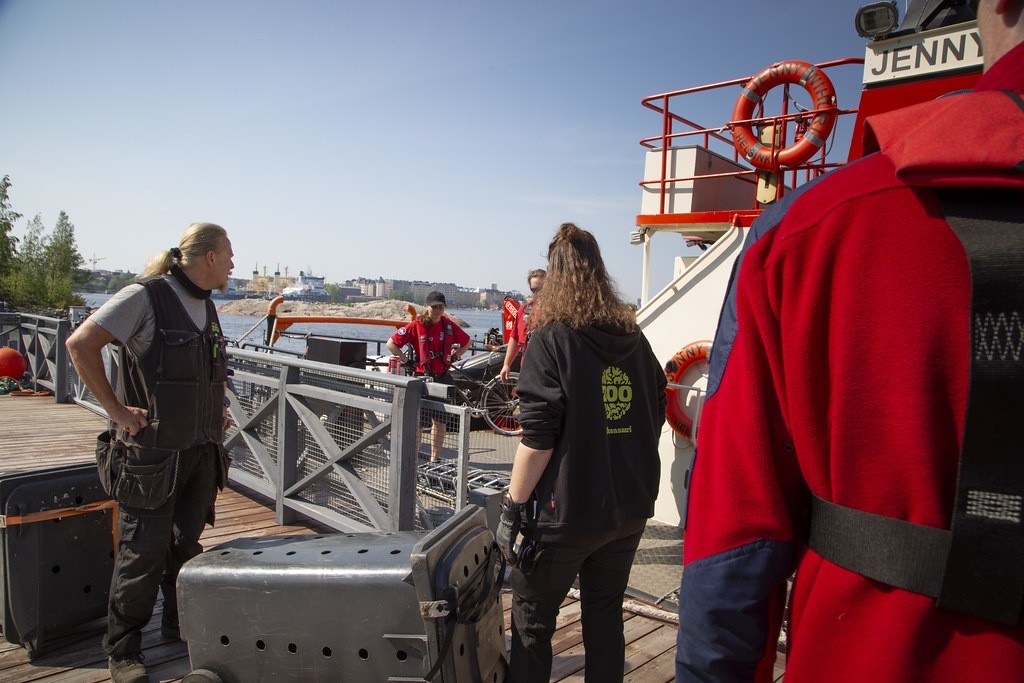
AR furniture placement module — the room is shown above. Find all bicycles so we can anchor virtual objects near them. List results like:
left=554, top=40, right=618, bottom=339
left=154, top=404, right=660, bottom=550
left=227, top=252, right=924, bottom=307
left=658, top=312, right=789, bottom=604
left=398, top=330, right=530, bottom=435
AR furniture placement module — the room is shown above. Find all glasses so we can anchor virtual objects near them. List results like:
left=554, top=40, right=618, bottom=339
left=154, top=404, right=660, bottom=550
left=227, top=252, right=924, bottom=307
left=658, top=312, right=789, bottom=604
left=530, top=288, right=541, bottom=293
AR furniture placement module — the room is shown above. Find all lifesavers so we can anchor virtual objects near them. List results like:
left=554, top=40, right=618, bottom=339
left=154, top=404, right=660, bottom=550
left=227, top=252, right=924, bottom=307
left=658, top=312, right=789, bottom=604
left=732, top=60, right=838, bottom=170
left=661, top=340, right=712, bottom=442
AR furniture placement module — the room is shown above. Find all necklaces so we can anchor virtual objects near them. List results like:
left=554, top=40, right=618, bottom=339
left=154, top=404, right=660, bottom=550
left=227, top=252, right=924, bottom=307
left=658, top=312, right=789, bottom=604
left=171, top=263, right=212, bottom=299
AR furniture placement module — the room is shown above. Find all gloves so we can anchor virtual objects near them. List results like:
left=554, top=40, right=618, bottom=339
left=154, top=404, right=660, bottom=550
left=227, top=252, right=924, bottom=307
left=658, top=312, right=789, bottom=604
left=495, top=497, right=523, bottom=565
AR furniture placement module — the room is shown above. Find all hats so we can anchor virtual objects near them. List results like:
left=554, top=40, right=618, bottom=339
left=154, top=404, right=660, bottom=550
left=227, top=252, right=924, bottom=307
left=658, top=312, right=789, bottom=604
left=426, top=290, right=447, bottom=307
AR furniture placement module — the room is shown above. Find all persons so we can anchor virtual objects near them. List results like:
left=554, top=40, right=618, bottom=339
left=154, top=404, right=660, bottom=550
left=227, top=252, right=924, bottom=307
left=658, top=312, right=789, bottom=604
left=495, top=327, right=499, bottom=334
left=499, top=270, right=546, bottom=384
left=494, top=221, right=667, bottom=682
left=668, top=1, right=1024, bottom=679
left=64, top=222, right=245, bottom=683
left=490, top=328, right=497, bottom=339
left=386, top=292, right=472, bottom=464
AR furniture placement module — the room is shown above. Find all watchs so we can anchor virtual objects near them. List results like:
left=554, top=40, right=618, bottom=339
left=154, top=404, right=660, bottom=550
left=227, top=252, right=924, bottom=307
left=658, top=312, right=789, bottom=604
left=224, top=396, right=230, bottom=407
left=502, top=493, right=527, bottom=512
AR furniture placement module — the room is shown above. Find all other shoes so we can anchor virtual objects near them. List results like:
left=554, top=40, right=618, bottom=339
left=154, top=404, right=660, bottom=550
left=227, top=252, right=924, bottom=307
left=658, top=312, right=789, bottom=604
left=106, top=655, right=149, bottom=683
